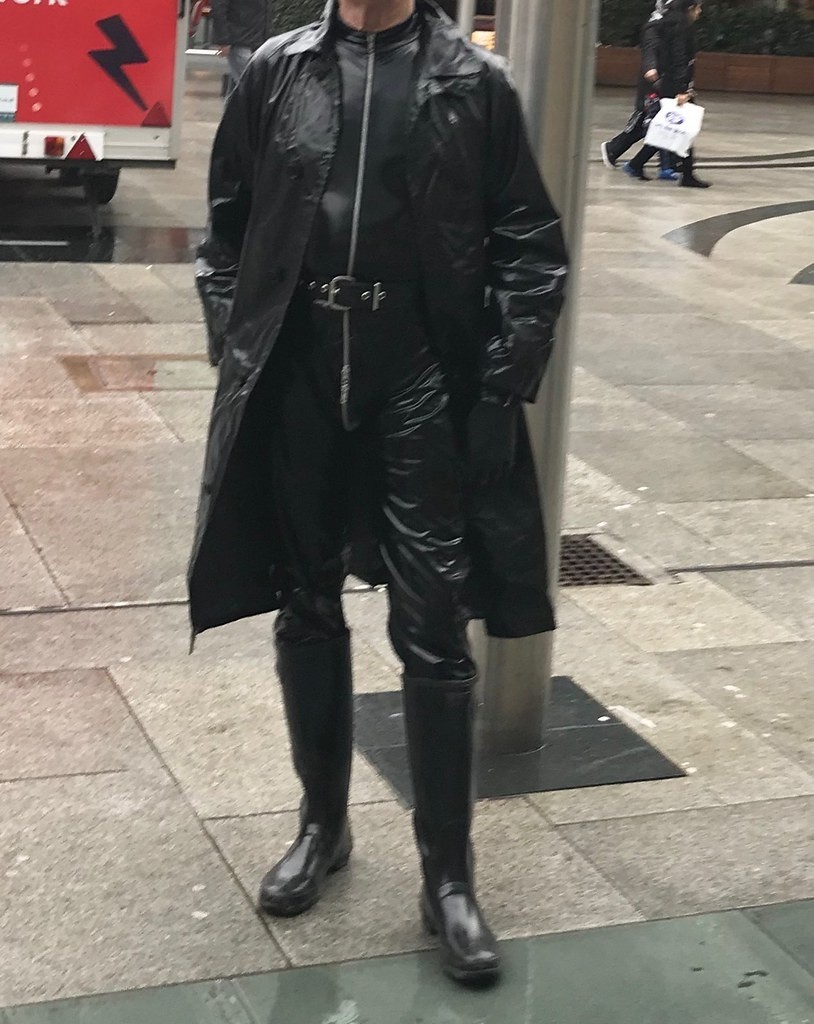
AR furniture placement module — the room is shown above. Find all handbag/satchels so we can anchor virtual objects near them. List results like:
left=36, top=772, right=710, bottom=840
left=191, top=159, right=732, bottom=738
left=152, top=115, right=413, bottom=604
left=643, top=98, right=706, bottom=159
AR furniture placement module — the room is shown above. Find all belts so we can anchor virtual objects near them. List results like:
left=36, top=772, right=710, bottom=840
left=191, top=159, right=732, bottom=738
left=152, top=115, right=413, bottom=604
left=295, top=275, right=424, bottom=314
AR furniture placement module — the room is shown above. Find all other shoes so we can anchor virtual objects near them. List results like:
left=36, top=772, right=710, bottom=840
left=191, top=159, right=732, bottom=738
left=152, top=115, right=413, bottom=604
left=680, top=175, right=713, bottom=188
left=621, top=161, right=646, bottom=180
left=658, top=168, right=679, bottom=180
left=600, top=141, right=617, bottom=170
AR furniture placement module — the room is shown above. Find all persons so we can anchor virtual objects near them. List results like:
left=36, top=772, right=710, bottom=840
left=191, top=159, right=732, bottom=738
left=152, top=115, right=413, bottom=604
left=600, top=0, right=713, bottom=188
left=213, top=0, right=275, bottom=84
left=185, top=0, right=573, bottom=982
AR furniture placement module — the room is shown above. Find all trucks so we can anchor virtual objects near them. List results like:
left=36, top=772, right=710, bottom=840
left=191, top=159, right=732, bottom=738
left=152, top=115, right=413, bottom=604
left=0, top=0, right=189, bottom=203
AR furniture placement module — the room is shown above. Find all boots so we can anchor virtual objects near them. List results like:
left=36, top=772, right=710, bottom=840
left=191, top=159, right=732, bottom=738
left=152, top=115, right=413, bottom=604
left=400, top=672, right=506, bottom=979
left=257, top=630, right=354, bottom=917
